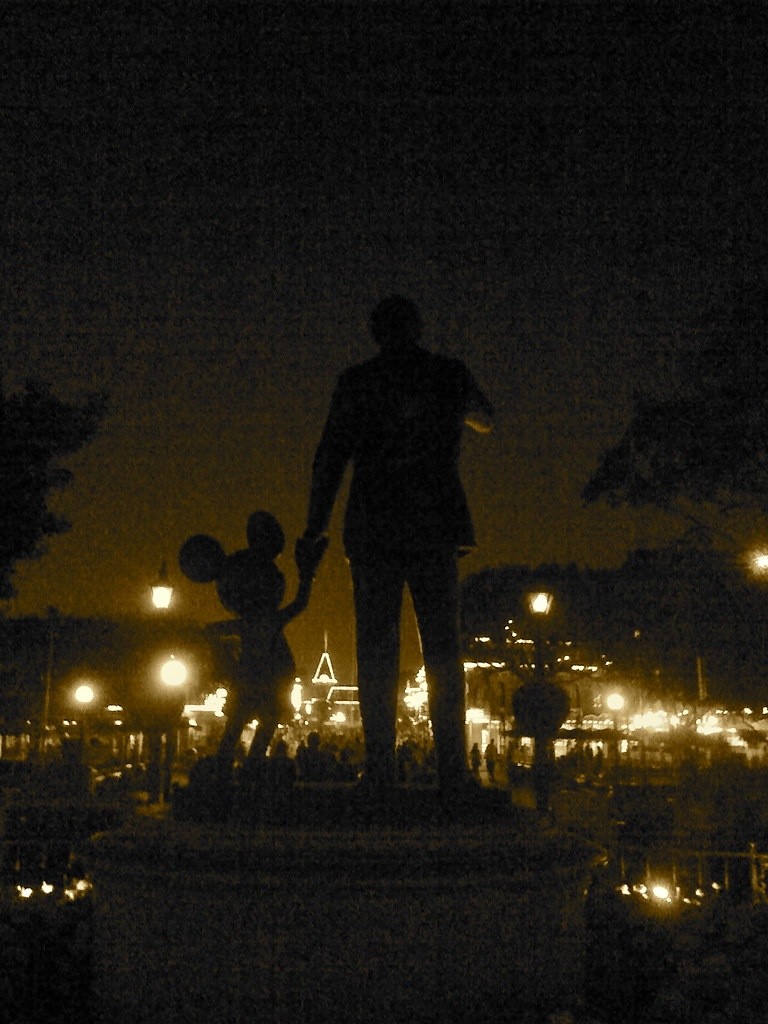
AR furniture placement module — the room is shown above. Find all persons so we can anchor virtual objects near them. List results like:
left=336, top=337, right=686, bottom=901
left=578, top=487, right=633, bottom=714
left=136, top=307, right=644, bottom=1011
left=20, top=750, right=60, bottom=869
left=180, top=731, right=605, bottom=781
left=296, top=295, right=498, bottom=798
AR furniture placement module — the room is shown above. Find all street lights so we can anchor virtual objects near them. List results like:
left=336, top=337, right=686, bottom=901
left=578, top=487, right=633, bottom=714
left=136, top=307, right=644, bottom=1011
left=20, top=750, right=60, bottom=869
left=518, top=578, right=554, bottom=816
